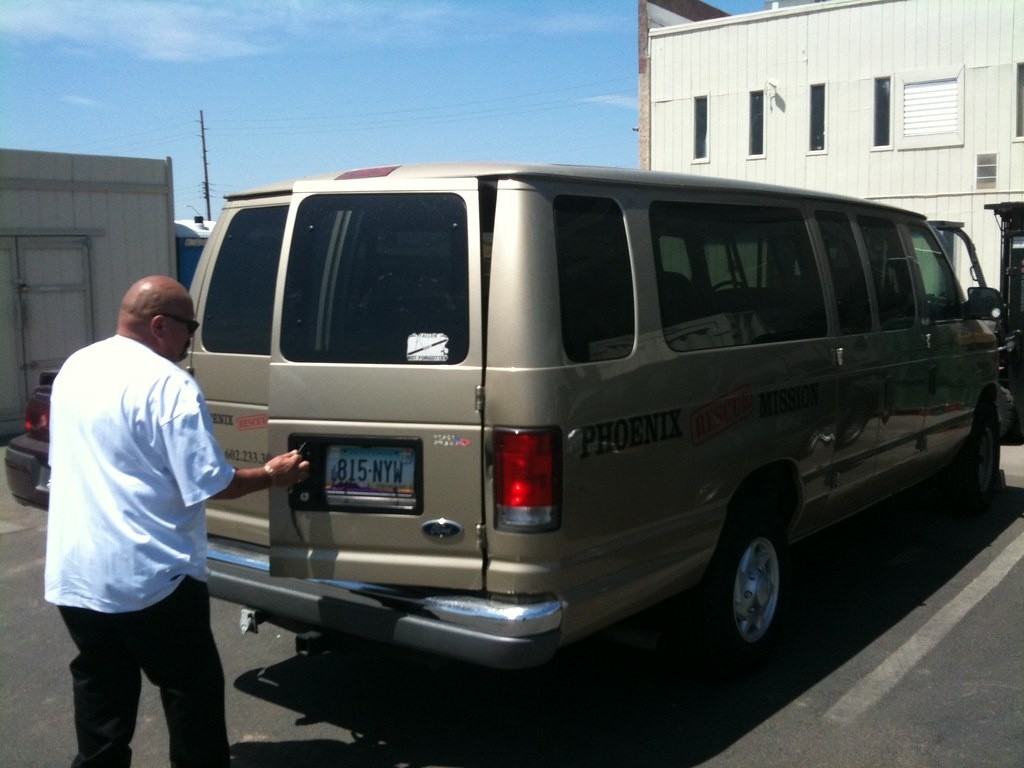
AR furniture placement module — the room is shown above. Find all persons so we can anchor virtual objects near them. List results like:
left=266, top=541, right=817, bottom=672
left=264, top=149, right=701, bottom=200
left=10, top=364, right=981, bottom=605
left=45, top=276, right=309, bottom=768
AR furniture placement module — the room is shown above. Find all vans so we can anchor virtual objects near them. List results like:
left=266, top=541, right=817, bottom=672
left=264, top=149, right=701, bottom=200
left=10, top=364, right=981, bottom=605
left=177, top=162, right=1003, bottom=669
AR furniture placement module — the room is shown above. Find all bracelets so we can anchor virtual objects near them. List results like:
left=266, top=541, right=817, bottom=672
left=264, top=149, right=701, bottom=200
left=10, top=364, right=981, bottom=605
left=264, top=466, right=274, bottom=487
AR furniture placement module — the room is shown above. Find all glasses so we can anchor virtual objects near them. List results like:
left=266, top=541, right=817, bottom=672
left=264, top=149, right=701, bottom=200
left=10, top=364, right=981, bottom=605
left=152, top=313, right=200, bottom=334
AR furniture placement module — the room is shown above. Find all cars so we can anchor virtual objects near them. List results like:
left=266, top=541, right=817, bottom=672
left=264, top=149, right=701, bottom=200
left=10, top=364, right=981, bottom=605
left=5, top=368, right=58, bottom=510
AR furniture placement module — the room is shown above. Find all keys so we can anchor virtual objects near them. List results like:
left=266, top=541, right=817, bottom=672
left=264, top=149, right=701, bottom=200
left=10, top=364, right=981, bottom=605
left=298, top=441, right=307, bottom=454
